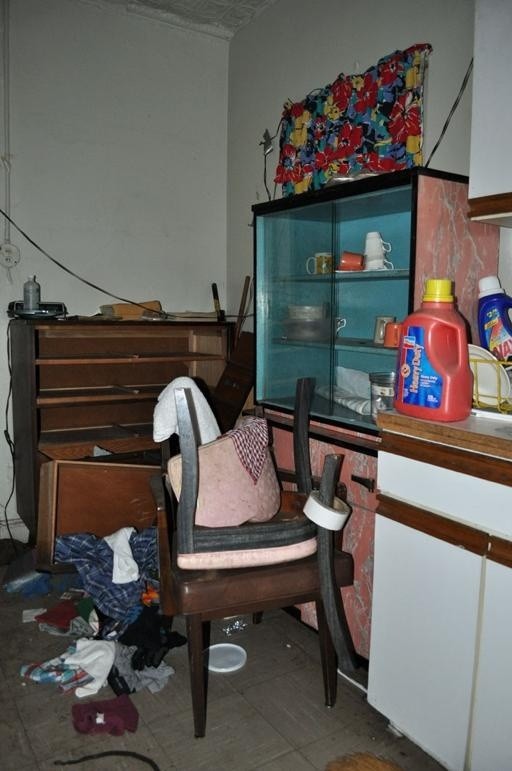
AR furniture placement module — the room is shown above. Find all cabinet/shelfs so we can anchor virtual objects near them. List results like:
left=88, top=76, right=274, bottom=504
left=11, top=276, right=254, bottom=574
left=365, top=435, right=492, bottom=771
left=469, top=436, right=511, bottom=771
left=251, top=167, right=500, bottom=667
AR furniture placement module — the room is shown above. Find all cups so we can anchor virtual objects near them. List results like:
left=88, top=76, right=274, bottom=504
left=374, top=317, right=403, bottom=348
left=306, top=232, right=394, bottom=275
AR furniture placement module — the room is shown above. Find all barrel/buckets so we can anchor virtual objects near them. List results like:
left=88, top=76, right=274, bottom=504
left=475, top=274, right=512, bottom=367
left=393, top=277, right=475, bottom=423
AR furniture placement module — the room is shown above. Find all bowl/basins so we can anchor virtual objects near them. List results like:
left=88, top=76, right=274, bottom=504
left=282, top=305, right=337, bottom=341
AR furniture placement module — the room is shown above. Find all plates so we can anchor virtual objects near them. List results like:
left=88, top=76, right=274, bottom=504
left=467, top=344, right=510, bottom=405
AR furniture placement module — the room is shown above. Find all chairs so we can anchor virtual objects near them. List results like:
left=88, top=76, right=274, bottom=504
left=150, top=384, right=366, bottom=741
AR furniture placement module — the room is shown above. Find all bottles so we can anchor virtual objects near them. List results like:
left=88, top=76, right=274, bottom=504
left=368, top=372, right=395, bottom=423
left=23, top=274, right=40, bottom=310
